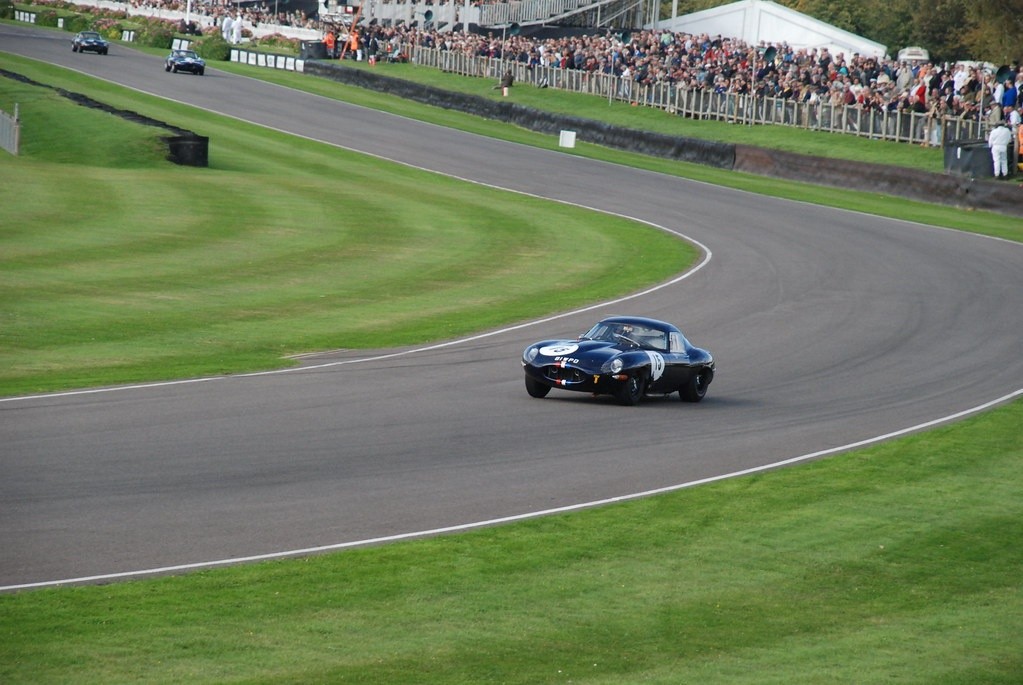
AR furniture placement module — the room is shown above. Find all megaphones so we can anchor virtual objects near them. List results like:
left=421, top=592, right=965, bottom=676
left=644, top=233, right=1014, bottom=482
left=757, top=46, right=777, bottom=61
left=990, top=65, right=1010, bottom=84
left=615, top=30, right=631, bottom=44
left=422, top=10, right=433, bottom=20
left=507, top=23, right=520, bottom=35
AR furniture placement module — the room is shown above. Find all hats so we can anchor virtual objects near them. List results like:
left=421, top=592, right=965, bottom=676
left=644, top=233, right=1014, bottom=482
left=990, top=101, right=997, bottom=104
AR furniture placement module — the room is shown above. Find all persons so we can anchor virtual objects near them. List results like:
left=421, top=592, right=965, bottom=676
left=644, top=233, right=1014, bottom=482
left=608, top=325, right=631, bottom=343
left=175, top=51, right=181, bottom=57
left=609, top=28, right=1023, bottom=175
left=322, top=24, right=409, bottom=67
left=190, top=0, right=276, bottom=27
left=987, top=120, right=1012, bottom=179
left=385, top=0, right=524, bottom=25
left=407, top=26, right=612, bottom=102
left=221, top=12, right=245, bottom=46
left=272, top=8, right=319, bottom=29
left=80, top=33, right=86, bottom=38
left=113, top=0, right=187, bottom=13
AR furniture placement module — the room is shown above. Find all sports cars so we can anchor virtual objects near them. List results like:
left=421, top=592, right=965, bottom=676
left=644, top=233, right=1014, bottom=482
left=521, top=317, right=717, bottom=406
left=165, top=50, right=206, bottom=76
left=71, top=31, right=109, bottom=55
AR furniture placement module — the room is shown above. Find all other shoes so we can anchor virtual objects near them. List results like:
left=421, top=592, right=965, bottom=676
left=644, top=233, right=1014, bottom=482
left=994, top=176, right=999, bottom=180
left=1002, top=175, right=1009, bottom=181
